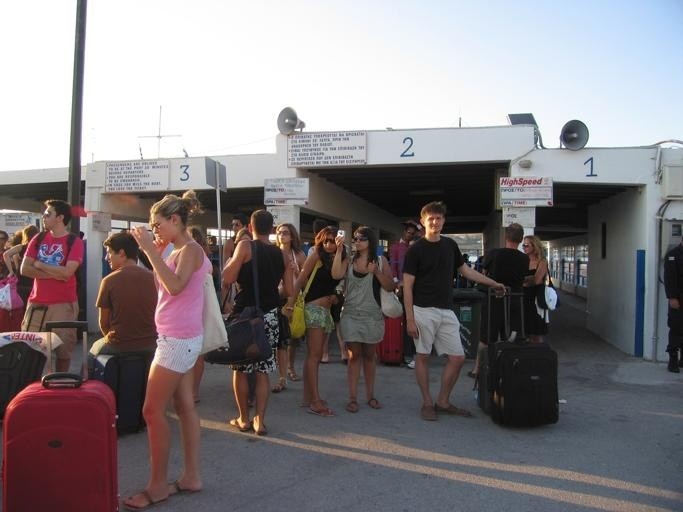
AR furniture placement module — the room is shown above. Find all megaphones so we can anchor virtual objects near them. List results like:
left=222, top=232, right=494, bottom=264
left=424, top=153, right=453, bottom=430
left=559, top=119, right=590, bottom=152
left=275, top=106, right=307, bottom=136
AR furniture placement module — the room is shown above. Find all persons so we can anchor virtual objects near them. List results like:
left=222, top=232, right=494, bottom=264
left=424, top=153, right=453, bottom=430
left=0, top=198, right=86, bottom=374
left=221, top=228, right=253, bottom=314
left=403, top=200, right=506, bottom=421
left=122, top=189, right=213, bottom=510
left=303, top=219, right=351, bottom=365
left=664, top=236, right=683, bottom=374
left=390, top=220, right=425, bottom=370
left=330, top=226, right=396, bottom=413
left=223, top=209, right=295, bottom=436
left=468, top=224, right=530, bottom=378
left=224, top=213, right=246, bottom=264
left=87, top=233, right=159, bottom=381
left=519, top=235, right=558, bottom=343
left=184, top=225, right=212, bottom=403
left=272, top=222, right=308, bottom=394
left=282, top=224, right=346, bottom=419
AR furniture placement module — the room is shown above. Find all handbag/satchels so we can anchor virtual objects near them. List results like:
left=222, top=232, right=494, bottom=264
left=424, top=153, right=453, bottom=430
left=282, top=294, right=306, bottom=340
left=201, top=271, right=229, bottom=356
left=380, top=288, right=403, bottom=317
left=0, top=275, right=24, bottom=309
left=205, top=304, right=272, bottom=365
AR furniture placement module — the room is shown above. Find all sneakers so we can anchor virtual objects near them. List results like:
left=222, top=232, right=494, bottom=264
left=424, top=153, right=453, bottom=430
left=467, top=371, right=476, bottom=377
left=408, top=361, right=415, bottom=368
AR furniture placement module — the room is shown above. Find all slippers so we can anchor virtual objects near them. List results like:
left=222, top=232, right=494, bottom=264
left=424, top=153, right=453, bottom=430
left=124, top=490, right=168, bottom=510
left=169, top=480, right=203, bottom=495
left=230, top=358, right=381, bottom=435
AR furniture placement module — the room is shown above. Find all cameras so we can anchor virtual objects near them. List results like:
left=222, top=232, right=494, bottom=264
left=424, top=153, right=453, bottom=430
left=337, top=229, right=345, bottom=238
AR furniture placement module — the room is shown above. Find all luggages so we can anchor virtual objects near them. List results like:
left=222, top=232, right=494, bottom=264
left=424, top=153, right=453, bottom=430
left=0, top=305, right=154, bottom=512
left=478, top=288, right=559, bottom=429
left=379, top=318, right=403, bottom=365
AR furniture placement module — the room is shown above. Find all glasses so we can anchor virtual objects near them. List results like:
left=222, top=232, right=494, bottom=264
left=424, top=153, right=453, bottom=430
left=151, top=223, right=161, bottom=230
left=231, top=222, right=415, bottom=243
left=44, top=210, right=54, bottom=218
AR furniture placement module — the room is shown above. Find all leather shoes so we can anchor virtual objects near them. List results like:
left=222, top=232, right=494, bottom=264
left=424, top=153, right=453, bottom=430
left=436, top=402, right=470, bottom=417
left=420, top=405, right=437, bottom=420
